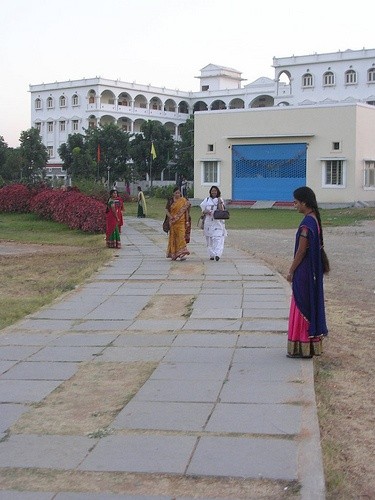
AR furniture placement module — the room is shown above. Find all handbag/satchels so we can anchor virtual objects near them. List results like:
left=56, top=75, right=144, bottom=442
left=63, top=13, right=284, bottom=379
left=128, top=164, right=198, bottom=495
left=197, top=214, right=206, bottom=229
left=308, top=213, right=330, bottom=273
left=213, top=203, right=230, bottom=219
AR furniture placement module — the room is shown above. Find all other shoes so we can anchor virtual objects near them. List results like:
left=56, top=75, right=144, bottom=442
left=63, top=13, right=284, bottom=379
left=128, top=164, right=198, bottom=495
left=210, top=258, right=214, bottom=260
left=216, top=256, right=219, bottom=261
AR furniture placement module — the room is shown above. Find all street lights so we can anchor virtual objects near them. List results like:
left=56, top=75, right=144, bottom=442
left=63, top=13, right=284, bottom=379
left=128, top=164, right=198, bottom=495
left=107, top=165, right=111, bottom=189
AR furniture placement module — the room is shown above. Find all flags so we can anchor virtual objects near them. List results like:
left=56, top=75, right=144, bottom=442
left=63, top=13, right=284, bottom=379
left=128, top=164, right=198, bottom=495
left=151, top=143, right=157, bottom=160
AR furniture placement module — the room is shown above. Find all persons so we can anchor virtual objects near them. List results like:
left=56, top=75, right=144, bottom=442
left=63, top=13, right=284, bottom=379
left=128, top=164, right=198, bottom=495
left=285, top=186, right=329, bottom=358
left=104, top=182, right=124, bottom=249
left=200, top=185, right=227, bottom=261
left=135, top=186, right=147, bottom=218
left=165, top=187, right=191, bottom=261
